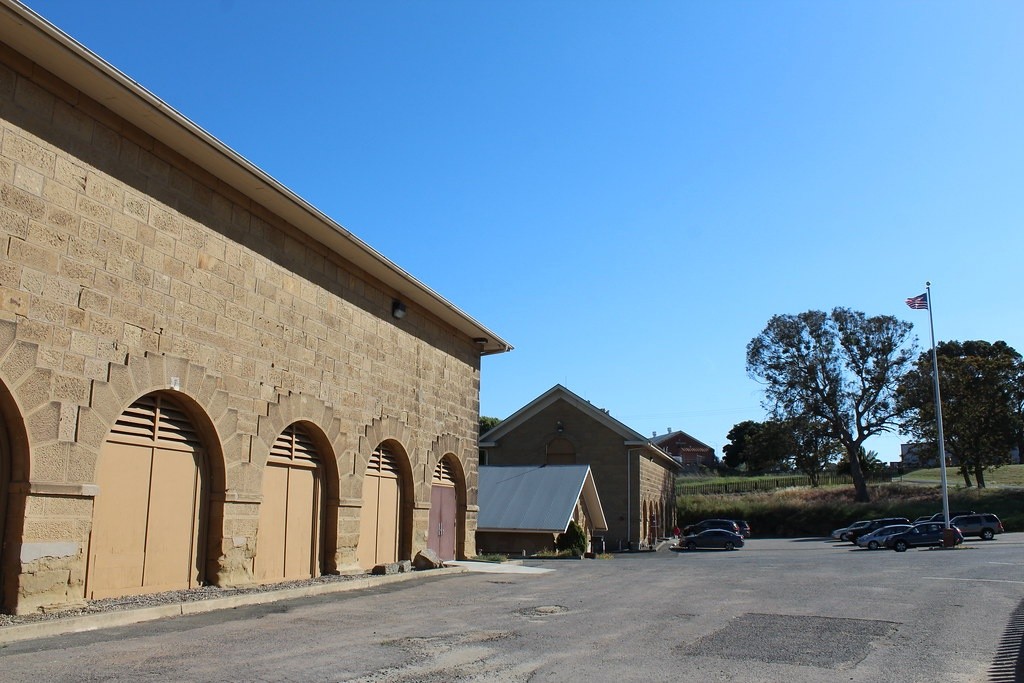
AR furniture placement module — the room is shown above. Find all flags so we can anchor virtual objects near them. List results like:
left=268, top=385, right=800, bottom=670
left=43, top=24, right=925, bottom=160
left=906, top=292, right=928, bottom=310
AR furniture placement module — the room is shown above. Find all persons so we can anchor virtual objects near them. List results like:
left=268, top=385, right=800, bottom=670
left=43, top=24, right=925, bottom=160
left=674, top=526, right=680, bottom=542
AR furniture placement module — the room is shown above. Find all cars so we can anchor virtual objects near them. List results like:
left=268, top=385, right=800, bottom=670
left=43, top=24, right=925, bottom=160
left=856, top=524, right=920, bottom=551
left=912, top=516, right=932, bottom=524
left=680, top=529, right=744, bottom=551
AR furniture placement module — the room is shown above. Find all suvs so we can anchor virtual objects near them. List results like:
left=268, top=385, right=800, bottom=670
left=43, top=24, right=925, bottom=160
left=732, top=520, right=751, bottom=536
left=884, top=522, right=965, bottom=553
left=950, top=512, right=1004, bottom=540
left=914, top=512, right=977, bottom=524
left=846, top=517, right=912, bottom=545
left=683, top=519, right=740, bottom=536
left=832, top=521, right=871, bottom=542
